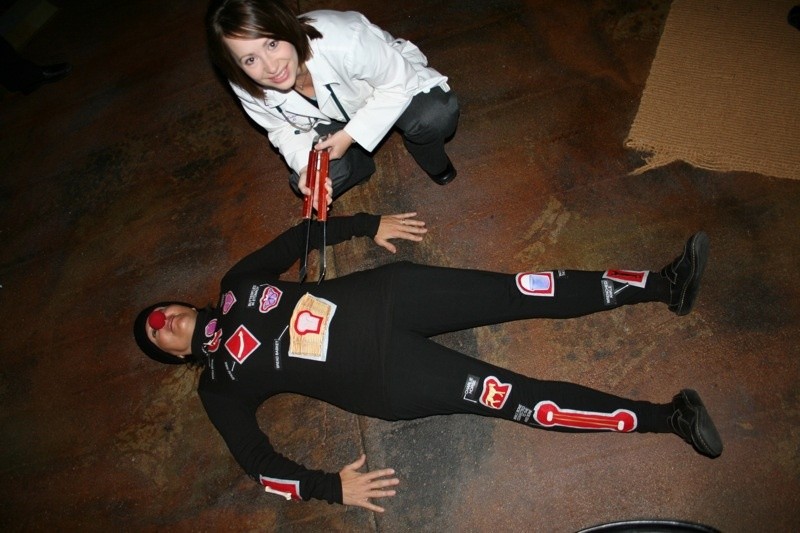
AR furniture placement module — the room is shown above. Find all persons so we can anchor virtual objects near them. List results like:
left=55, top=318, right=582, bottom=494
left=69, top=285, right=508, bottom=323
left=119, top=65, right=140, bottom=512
left=206, top=0, right=460, bottom=200
left=133, top=212, right=723, bottom=512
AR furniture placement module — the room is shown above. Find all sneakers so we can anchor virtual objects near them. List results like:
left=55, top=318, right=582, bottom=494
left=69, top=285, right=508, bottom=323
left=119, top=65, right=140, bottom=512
left=659, top=230, right=710, bottom=317
left=667, top=389, right=724, bottom=459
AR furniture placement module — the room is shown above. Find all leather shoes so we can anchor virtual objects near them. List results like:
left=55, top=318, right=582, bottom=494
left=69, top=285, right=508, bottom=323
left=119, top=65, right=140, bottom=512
left=412, top=149, right=458, bottom=185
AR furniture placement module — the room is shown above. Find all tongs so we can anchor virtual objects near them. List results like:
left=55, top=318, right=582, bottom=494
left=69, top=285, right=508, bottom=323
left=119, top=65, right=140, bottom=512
left=299, top=135, right=329, bottom=285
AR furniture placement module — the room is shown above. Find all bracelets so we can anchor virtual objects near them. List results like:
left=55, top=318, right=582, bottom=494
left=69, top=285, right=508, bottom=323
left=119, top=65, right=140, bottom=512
left=296, top=70, right=308, bottom=90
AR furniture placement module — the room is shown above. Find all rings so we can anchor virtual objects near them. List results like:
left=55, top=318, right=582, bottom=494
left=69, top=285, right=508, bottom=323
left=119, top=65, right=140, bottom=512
left=402, top=219, right=405, bottom=225
